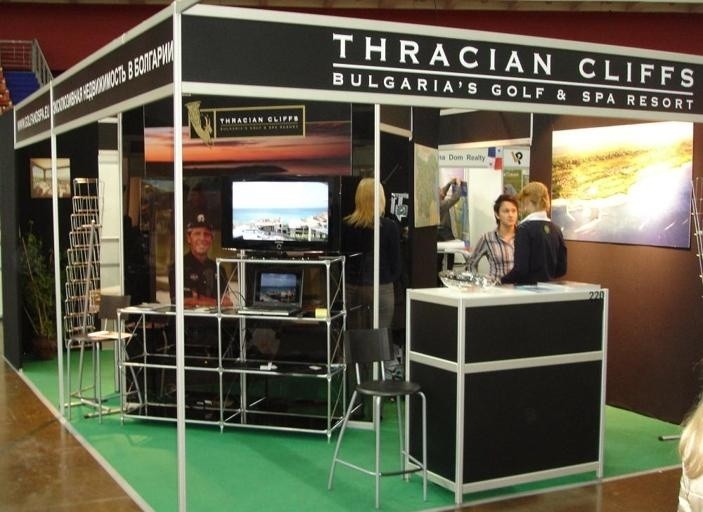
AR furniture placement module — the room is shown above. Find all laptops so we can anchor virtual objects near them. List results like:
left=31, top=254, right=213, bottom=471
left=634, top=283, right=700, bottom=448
left=238, top=268, right=304, bottom=316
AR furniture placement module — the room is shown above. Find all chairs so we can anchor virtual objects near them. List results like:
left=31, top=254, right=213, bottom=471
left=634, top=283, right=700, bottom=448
left=61, top=292, right=140, bottom=421
left=322, top=326, right=435, bottom=511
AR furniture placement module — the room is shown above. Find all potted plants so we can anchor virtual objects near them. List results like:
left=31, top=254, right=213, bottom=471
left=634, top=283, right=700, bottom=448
left=8, top=220, right=62, bottom=370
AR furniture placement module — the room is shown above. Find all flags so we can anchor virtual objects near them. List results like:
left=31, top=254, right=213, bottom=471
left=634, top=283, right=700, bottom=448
left=485, top=145, right=504, bottom=171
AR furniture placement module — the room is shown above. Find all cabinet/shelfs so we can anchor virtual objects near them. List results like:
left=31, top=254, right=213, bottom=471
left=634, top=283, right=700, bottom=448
left=400, top=278, right=615, bottom=506
left=60, top=173, right=101, bottom=353
left=115, top=251, right=354, bottom=441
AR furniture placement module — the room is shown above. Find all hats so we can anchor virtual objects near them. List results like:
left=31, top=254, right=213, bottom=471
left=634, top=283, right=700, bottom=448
left=187, top=208, right=212, bottom=230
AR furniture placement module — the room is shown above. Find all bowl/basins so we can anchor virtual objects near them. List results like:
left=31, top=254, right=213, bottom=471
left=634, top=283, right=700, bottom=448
left=438, top=269, right=496, bottom=292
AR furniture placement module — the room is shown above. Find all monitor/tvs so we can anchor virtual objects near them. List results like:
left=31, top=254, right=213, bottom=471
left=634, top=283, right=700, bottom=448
left=221, top=173, right=342, bottom=260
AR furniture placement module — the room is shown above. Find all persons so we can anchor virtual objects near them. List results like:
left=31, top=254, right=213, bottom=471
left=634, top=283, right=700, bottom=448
left=435, top=175, right=464, bottom=274
left=501, top=180, right=569, bottom=286
left=675, top=398, right=703, bottom=511
left=167, top=211, right=236, bottom=360
left=338, top=176, right=403, bottom=423
left=468, top=193, right=521, bottom=283
